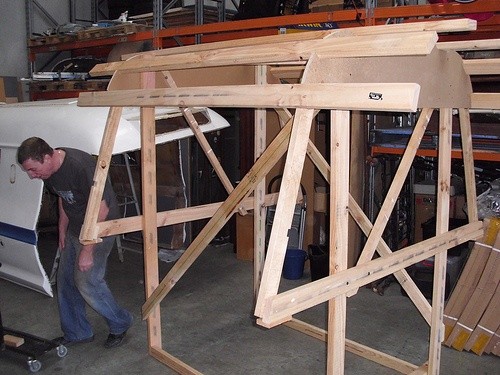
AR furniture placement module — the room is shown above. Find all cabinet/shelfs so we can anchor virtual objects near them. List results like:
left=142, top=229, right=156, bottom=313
left=26, top=0, right=499, bottom=296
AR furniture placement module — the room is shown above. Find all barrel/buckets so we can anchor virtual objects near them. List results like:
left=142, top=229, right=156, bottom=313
left=283, top=249, right=308, bottom=280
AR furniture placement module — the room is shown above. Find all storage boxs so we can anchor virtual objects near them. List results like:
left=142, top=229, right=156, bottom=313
left=412, top=182, right=500, bottom=357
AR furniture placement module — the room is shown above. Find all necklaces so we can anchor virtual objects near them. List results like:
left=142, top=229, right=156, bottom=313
left=58, top=150, right=62, bottom=166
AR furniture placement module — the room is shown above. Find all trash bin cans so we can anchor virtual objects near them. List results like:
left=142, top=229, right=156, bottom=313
left=308, top=243, right=330, bottom=281
left=282, top=249, right=308, bottom=280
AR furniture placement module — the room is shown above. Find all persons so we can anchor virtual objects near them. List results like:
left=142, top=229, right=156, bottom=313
left=17, top=137, right=137, bottom=348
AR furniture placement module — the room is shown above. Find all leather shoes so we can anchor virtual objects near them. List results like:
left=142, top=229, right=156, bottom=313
left=52, top=334, right=95, bottom=346
left=103, top=331, right=127, bottom=349
